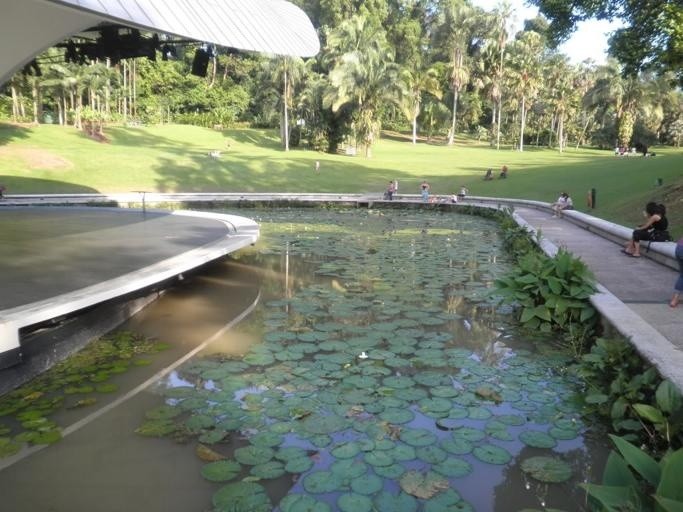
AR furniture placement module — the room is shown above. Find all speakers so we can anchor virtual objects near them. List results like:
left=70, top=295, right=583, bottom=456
left=191, top=49, right=210, bottom=78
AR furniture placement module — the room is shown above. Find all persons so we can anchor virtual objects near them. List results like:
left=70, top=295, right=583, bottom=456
left=386, top=180, right=468, bottom=204
left=669, top=237, right=683, bottom=309
left=620, top=202, right=669, bottom=257
left=614, top=145, right=637, bottom=159
left=552, top=192, right=574, bottom=219
left=500, top=165, right=508, bottom=177
left=485, top=168, right=493, bottom=180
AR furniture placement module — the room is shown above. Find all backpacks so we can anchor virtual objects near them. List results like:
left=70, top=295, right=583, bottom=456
left=646, top=234, right=674, bottom=253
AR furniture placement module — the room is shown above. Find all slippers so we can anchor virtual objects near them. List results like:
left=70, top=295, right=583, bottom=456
left=629, top=254, right=641, bottom=258
left=669, top=299, right=679, bottom=308
left=620, top=249, right=632, bottom=255
